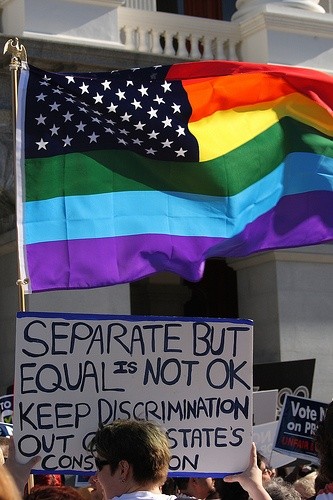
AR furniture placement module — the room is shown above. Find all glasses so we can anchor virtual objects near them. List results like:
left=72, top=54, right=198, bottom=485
left=95, top=457, right=115, bottom=471
left=263, top=466, right=270, bottom=475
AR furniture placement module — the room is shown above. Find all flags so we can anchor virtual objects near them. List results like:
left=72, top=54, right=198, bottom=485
left=16, top=60, right=333, bottom=295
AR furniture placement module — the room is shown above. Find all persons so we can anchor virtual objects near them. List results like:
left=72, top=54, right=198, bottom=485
left=0, top=409, right=333, bottom=500
left=174, top=476, right=216, bottom=500
